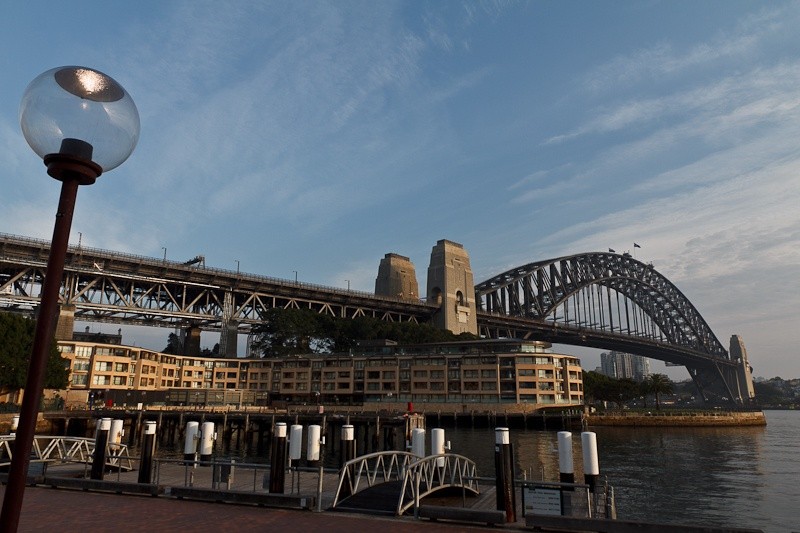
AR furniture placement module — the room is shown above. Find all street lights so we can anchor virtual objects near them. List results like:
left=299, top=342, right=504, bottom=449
left=162, top=247, right=167, bottom=260
left=387, top=392, right=393, bottom=412
left=235, top=260, right=239, bottom=272
left=293, top=271, right=297, bottom=281
left=345, top=280, right=350, bottom=291
left=315, top=392, right=321, bottom=410
left=1, top=67, right=140, bottom=533
left=195, top=393, right=199, bottom=409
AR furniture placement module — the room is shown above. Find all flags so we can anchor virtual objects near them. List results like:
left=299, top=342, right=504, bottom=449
left=634, top=243, right=640, bottom=248
